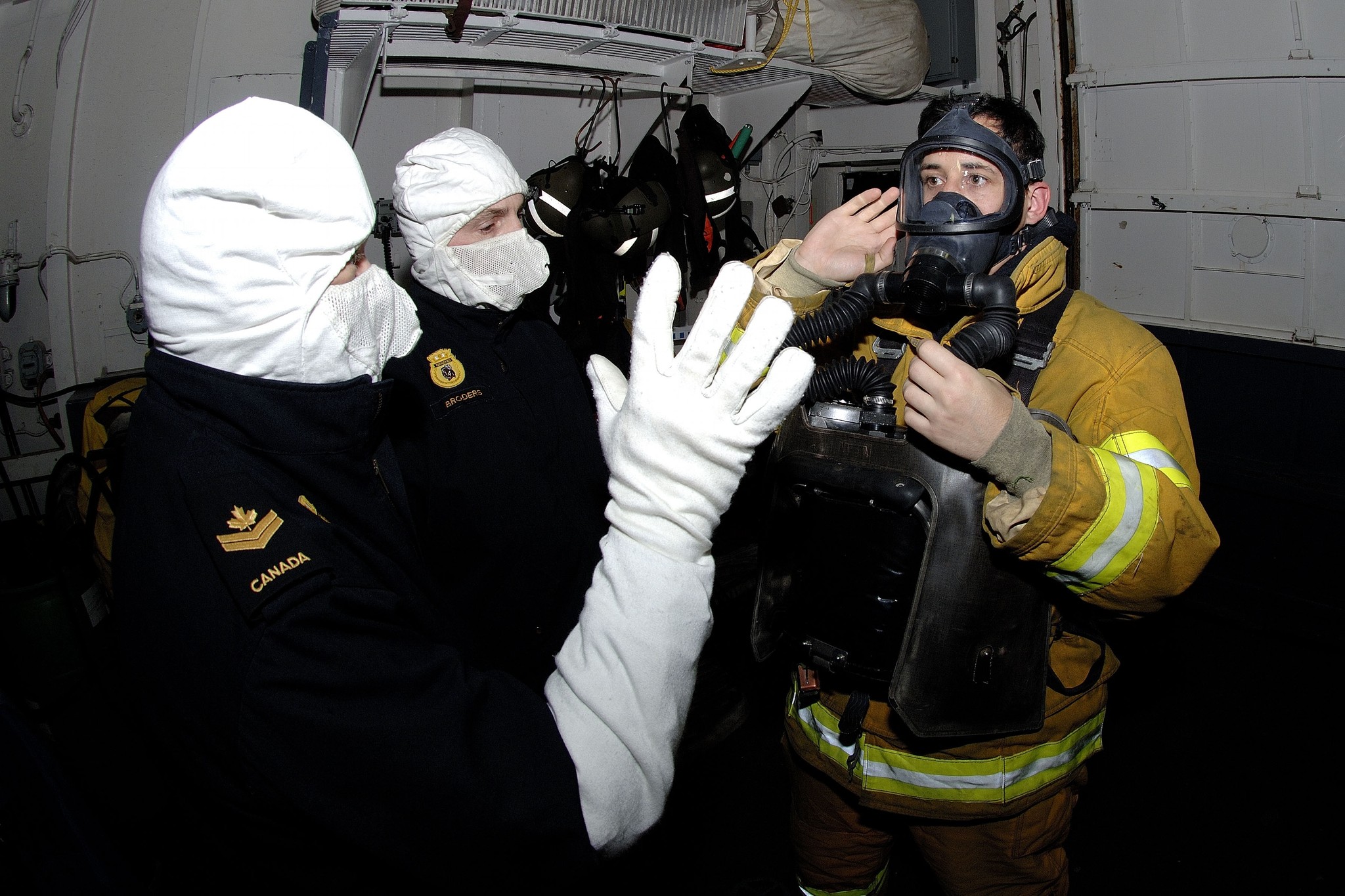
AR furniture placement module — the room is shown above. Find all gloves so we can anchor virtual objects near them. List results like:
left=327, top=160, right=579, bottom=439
left=541, top=247, right=823, bottom=859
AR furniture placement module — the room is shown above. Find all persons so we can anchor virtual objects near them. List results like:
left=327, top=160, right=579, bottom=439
left=719, top=91, right=1222, bottom=895
left=382, top=127, right=717, bottom=896
left=113, top=95, right=817, bottom=896
